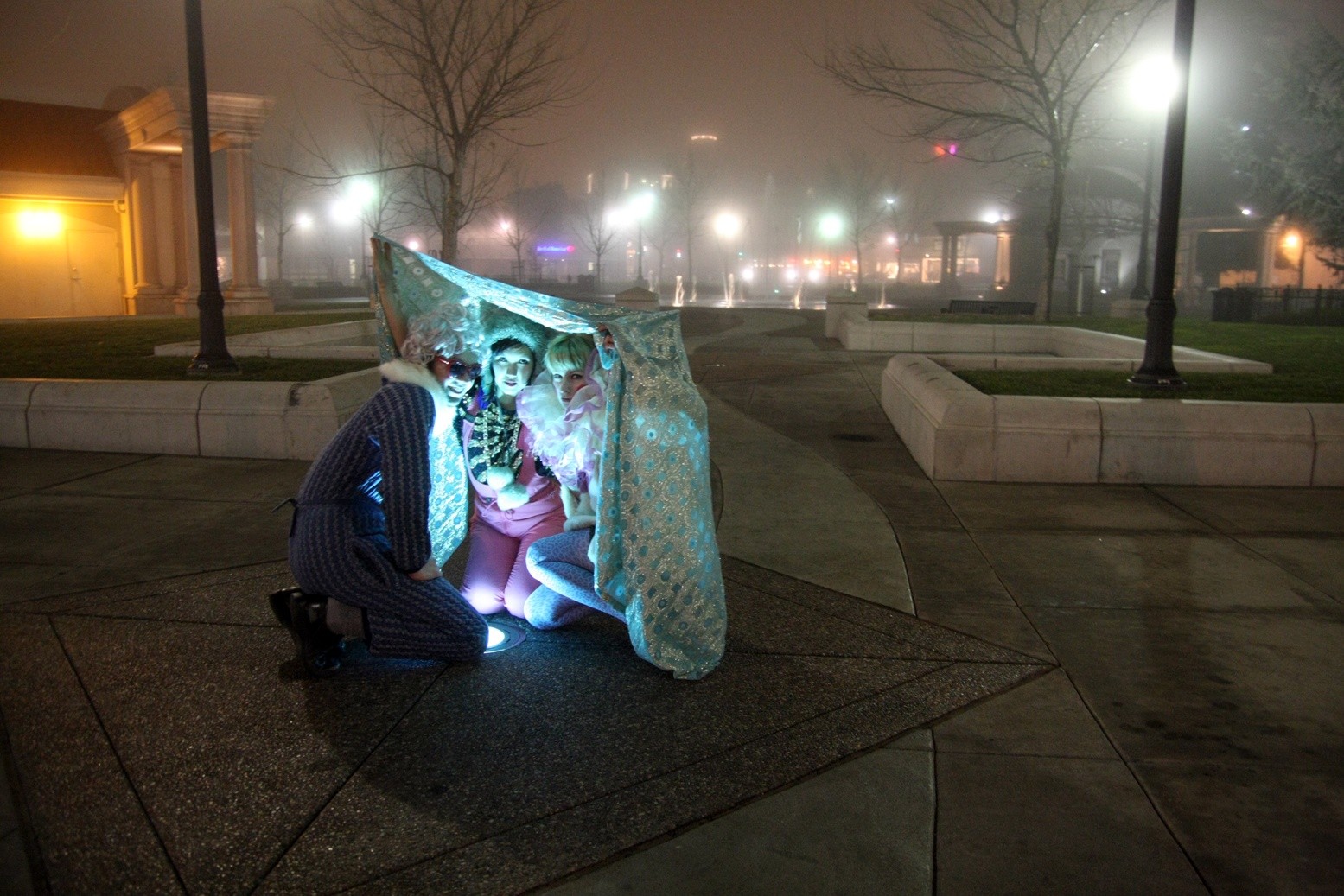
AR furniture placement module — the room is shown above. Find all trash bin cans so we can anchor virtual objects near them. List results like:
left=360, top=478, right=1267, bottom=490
left=1211, top=287, right=1236, bottom=323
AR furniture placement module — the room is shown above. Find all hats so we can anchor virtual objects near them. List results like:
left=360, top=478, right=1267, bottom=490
left=479, top=312, right=545, bottom=395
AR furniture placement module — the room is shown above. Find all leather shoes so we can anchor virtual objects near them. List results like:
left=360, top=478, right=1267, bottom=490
left=269, top=587, right=348, bottom=667
left=289, top=592, right=341, bottom=678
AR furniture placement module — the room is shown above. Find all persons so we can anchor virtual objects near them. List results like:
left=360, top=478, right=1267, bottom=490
left=516, top=324, right=628, bottom=630
left=267, top=304, right=489, bottom=682
left=369, top=234, right=564, bottom=619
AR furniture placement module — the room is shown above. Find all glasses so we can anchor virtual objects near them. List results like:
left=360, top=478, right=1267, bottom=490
left=433, top=354, right=485, bottom=381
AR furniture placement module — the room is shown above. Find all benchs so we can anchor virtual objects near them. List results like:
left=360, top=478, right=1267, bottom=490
left=941, top=299, right=1036, bottom=316
